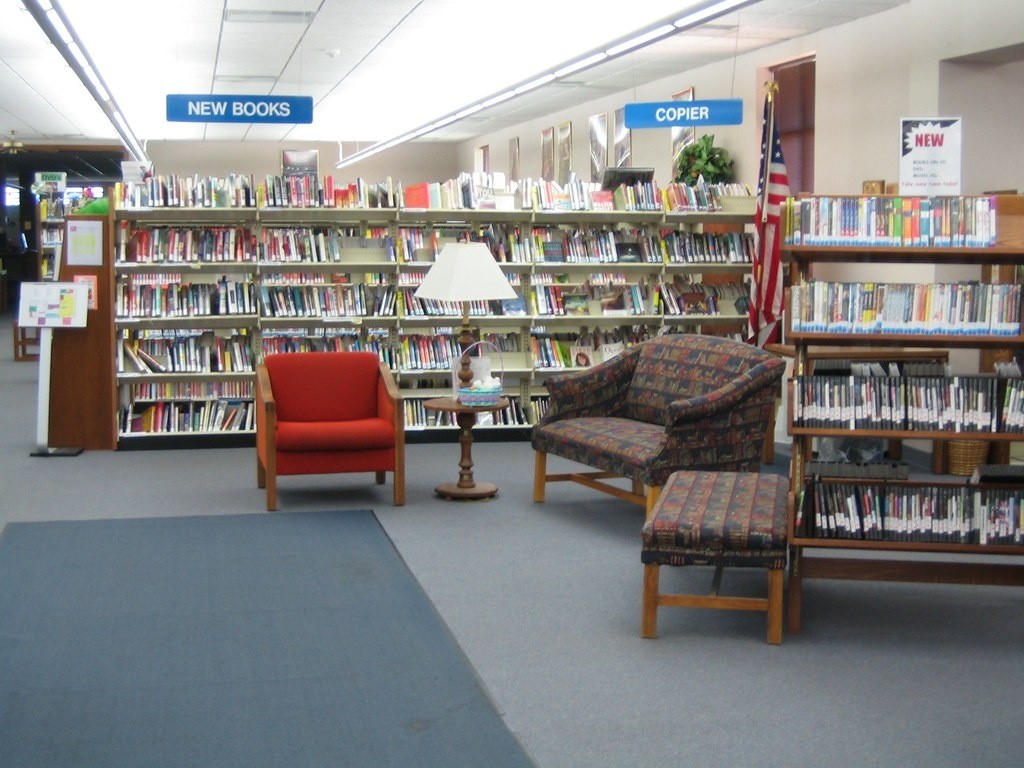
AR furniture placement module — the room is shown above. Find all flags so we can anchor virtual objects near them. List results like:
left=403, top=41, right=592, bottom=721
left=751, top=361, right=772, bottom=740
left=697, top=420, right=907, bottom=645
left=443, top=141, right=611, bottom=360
left=743, top=93, right=791, bottom=351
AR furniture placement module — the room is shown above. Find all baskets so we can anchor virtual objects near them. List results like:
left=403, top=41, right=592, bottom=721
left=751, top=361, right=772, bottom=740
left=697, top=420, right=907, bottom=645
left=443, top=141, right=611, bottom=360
left=457, top=341, right=504, bottom=406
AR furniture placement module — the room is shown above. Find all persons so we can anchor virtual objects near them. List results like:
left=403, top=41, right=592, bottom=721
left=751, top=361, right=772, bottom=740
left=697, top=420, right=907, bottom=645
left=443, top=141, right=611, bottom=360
left=573, top=352, right=592, bottom=368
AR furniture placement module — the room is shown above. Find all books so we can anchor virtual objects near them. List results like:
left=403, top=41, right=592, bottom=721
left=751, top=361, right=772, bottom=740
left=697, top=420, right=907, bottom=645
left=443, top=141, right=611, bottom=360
left=793, top=355, right=1024, bottom=434
left=118, top=326, right=748, bottom=370
left=783, top=194, right=998, bottom=247
left=794, top=473, right=1023, bottom=546
left=117, top=217, right=761, bottom=270
left=120, top=149, right=755, bottom=213
left=118, top=274, right=751, bottom=320
left=122, top=374, right=548, bottom=433
left=795, top=277, right=1023, bottom=341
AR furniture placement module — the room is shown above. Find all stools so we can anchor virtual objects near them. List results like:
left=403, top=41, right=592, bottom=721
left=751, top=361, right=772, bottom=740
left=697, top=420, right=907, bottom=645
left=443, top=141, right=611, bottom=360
left=641, top=470, right=789, bottom=644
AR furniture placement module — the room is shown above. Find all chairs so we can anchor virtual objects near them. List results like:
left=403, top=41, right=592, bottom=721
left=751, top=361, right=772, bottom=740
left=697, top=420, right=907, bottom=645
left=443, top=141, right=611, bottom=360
left=255, top=351, right=405, bottom=511
left=531, top=334, right=787, bottom=521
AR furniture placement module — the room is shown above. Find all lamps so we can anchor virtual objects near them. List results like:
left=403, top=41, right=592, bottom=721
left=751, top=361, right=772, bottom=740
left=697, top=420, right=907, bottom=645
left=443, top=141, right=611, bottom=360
left=413, top=242, right=518, bottom=403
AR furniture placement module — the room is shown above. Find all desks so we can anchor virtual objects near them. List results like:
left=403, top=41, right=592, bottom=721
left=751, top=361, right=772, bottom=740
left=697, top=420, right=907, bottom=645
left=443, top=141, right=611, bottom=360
left=424, top=397, right=510, bottom=501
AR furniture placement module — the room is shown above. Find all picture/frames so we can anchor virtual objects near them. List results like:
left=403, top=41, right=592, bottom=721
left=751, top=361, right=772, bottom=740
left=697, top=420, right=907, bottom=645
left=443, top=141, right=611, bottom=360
left=589, top=113, right=608, bottom=183
left=558, top=121, right=572, bottom=191
left=671, top=87, right=695, bottom=154
left=614, top=106, right=632, bottom=167
left=281, top=149, right=320, bottom=183
left=509, top=137, right=519, bottom=180
left=542, top=127, right=554, bottom=181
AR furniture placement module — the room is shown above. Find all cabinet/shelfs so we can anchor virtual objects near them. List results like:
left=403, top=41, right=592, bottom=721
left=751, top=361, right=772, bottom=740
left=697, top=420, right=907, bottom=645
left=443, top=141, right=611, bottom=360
left=48, top=216, right=111, bottom=450
left=780, top=193, right=1024, bottom=635
left=110, top=192, right=758, bottom=450
left=37, top=192, right=110, bottom=284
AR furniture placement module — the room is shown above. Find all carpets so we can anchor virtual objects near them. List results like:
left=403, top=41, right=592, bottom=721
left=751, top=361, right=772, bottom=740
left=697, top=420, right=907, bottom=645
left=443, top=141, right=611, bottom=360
left=0, top=510, right=535, bottom=768
